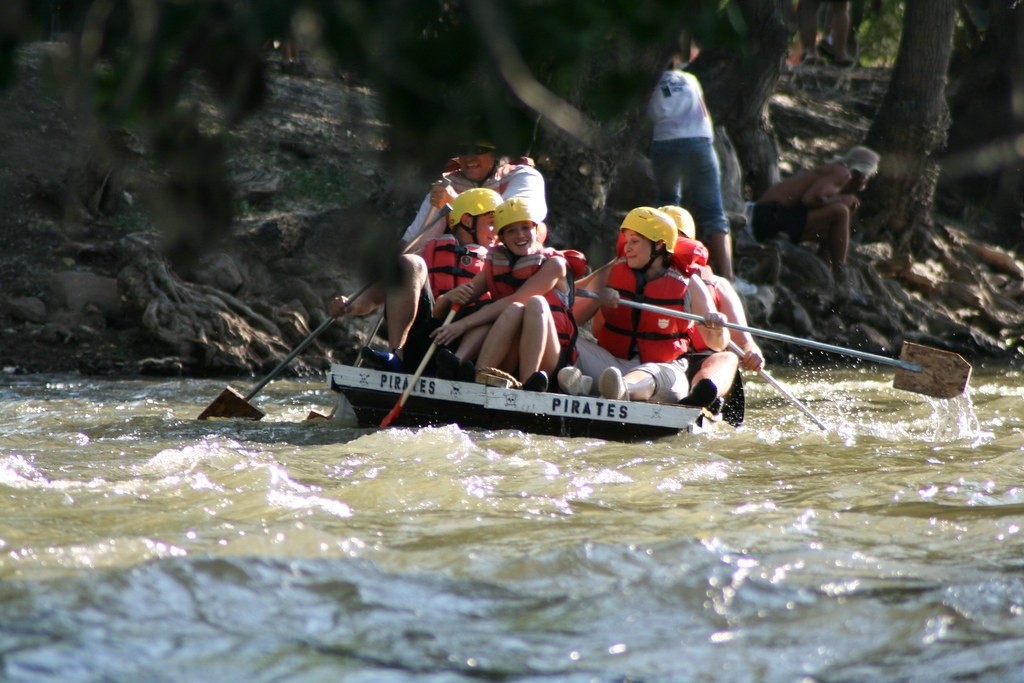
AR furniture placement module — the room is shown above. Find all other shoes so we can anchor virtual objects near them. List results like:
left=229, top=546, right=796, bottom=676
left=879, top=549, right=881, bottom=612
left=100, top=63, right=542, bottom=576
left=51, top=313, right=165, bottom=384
left=829, top=51, right=855, bottom=66
left=732, top=278, right=758, bottom=296
left=818, top=39, right=833, bottom=57
left=361, top=345, right=404, bottom=373
left=803, top=53, right=828, bottom=66
left=524, top=371, right=548, bottom=392
left=435, top=349, right=465, bottom=382
left=599, top=367, right=627, bottom=400
left=678, top=379, right=717, bottom=406
left=558, top=367, right=594, bottom=396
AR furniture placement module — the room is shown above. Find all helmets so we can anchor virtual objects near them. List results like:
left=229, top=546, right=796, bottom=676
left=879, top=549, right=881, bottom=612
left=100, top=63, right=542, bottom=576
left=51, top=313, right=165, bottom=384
left=450, top=188, right=504, bottom=228
left=620, top=206, right=677, bottom=253
left=495, top=199, right=539, bottom=234
left=456, top=116, right=499, bottom=149
left=658, top=206, right=696, bottom=240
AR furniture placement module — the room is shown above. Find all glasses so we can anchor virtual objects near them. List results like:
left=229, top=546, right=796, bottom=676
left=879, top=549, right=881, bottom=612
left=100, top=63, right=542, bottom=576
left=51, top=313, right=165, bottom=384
left=460, top=148, right=492, bottom=155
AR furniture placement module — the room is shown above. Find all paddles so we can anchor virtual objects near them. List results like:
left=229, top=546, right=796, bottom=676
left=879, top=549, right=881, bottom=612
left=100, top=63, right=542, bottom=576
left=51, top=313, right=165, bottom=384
left=306, top=177, right=453, bottom=422
left=724, top=333, right=830, bottom=433
left=196, top=200, right=455, bottom=420
left=376, top=298, right=464, bottom=429
left=576, top=286, right=973, bottom=401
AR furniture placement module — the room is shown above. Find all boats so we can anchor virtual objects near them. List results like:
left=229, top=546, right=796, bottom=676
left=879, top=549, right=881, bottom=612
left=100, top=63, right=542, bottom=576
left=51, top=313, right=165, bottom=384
left=326, top=361, right=704, bottom=444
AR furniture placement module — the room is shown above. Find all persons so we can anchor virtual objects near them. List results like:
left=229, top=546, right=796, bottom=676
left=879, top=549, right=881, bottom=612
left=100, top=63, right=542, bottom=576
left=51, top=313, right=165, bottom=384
left=648, top=59, right=759, bottom=297
left=329, top=137, right=765, bottom=410
left=751, top=145, right=879, bottom=272
left=795, top=1, right=863, bottom=71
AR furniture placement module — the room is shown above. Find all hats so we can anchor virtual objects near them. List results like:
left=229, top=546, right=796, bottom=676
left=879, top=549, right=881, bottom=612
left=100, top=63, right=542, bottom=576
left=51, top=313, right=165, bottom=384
left=847, top=147, right=880, bottom=175
